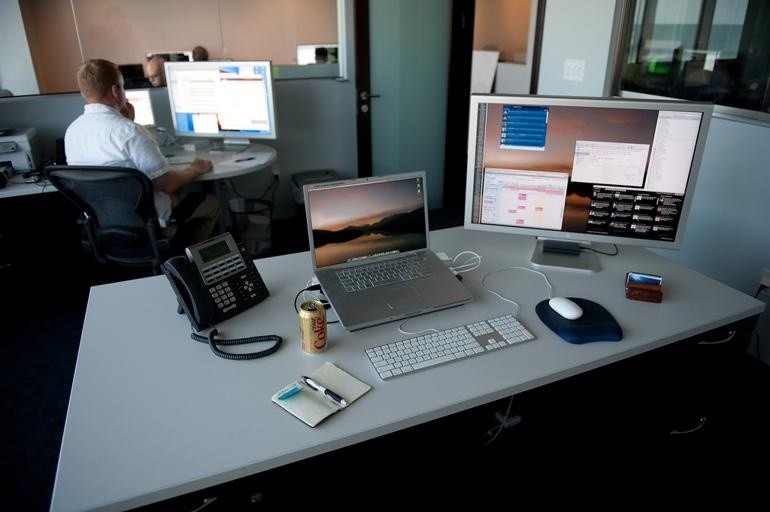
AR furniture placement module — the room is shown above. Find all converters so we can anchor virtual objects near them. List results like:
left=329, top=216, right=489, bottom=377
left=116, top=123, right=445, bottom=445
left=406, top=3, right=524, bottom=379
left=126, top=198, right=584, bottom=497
left=183, top=144, right=196, bottom=152
left=436, top=252, right=452, bottom=266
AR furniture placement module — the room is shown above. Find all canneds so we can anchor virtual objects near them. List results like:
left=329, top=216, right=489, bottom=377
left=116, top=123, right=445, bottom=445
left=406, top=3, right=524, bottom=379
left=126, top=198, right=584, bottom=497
left=298, top=300, right=328, bottom=356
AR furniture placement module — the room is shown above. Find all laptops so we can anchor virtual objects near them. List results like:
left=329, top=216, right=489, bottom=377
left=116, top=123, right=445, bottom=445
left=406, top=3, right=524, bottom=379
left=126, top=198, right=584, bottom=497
left=303, top=170, right=474, bottom=331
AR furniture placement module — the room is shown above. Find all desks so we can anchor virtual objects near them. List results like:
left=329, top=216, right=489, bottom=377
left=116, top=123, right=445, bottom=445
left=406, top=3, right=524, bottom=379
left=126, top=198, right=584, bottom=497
left=50, top=227, right=766, bottom=512
left=0, top=139, right=279, bottom=234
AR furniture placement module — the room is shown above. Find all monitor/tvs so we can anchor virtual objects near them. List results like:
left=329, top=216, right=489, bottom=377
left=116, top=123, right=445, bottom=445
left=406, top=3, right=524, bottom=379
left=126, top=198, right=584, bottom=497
left=164, top=60, right=279, bottom=153
left=118, top=64, right=145, bottom=80
left=148, top=51, right=194, bottom=61
left=295, top=44, right=337, bottom=65
left=463, top=93, right=714, bottom=273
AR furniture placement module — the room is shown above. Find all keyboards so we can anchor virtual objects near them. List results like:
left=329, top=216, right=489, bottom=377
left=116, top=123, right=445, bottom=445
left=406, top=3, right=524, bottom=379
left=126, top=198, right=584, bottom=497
left=364, top=313, right=537, bottom=381
left=165, top=156, right=194, bottom=165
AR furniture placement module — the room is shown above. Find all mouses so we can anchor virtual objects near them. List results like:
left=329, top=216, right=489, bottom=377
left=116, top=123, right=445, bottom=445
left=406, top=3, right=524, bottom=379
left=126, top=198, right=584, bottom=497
left=548, top=296, right=583, bottom=320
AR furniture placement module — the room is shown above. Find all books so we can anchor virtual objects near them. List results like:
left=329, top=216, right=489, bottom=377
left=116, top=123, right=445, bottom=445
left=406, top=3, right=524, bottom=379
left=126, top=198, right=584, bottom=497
left=272, top=360, right=373, bottom=429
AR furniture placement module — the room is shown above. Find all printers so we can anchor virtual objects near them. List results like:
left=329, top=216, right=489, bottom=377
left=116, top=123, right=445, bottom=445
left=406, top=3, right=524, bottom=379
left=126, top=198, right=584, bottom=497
left=0, top=127, right=44, bottom=189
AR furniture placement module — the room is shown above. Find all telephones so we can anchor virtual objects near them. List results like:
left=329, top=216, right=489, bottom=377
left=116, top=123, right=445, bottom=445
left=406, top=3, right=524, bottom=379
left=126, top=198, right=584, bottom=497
left=160, top=228, right=283, bottom=335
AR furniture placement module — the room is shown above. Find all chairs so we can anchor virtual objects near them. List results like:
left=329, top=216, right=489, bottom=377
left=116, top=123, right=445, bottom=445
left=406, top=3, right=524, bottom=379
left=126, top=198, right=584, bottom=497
left=45, top=165, right=179, bottom=275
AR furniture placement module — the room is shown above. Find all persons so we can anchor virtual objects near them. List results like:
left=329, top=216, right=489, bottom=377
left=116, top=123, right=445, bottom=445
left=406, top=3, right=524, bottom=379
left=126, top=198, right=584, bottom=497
left=64, top=58, right=223, bottom=250
left=146, top=58, right=167, bottom=88
left=193, top=47, right=211, bottom=62
left=310, top=48, right=331, bottom=64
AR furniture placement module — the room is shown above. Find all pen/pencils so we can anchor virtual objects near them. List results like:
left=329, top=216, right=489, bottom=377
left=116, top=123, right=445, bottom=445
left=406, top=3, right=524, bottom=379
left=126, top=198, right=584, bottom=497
left=236, top=158, right=256, bottom=162
left=300, top=374, right=349, bottom=408
